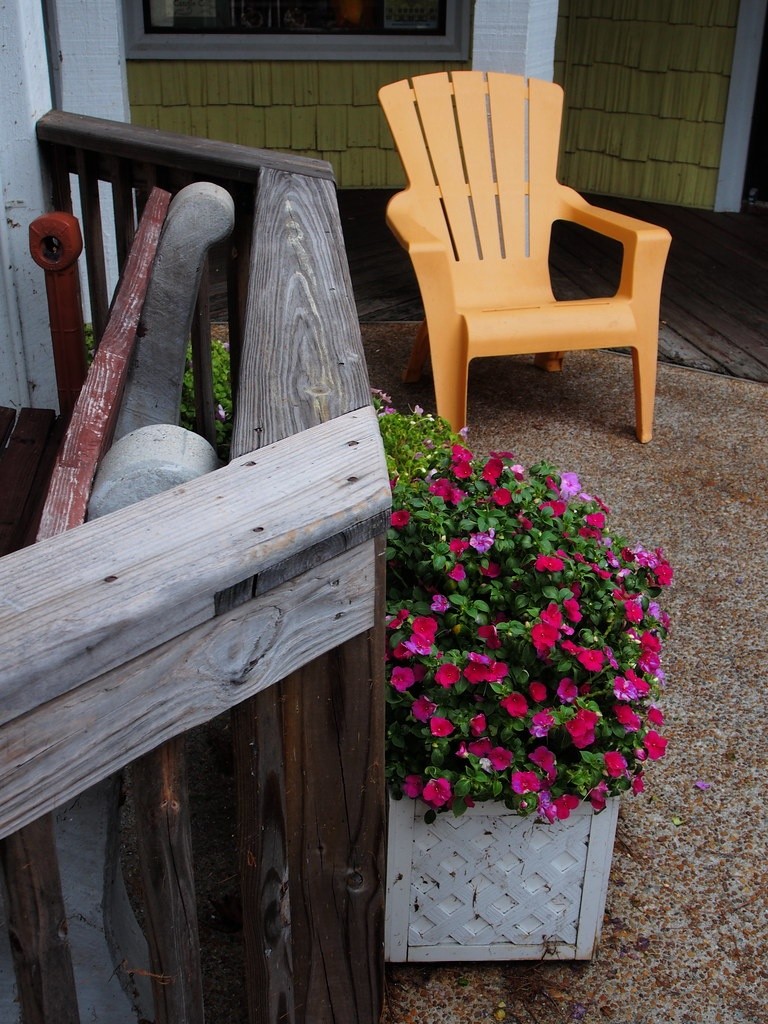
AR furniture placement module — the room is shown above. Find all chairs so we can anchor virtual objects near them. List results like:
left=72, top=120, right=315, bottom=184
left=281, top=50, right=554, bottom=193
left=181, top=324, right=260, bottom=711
left=373, top=69, right=672, bottom=444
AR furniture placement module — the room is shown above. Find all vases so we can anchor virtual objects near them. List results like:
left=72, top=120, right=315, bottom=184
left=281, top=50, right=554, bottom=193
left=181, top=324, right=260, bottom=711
left=380, top=787, right=622, bottom=965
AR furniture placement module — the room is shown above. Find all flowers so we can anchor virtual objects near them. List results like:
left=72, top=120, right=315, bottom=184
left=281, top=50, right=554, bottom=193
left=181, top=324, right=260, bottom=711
left=82, top=321, right=674, bottom=830
